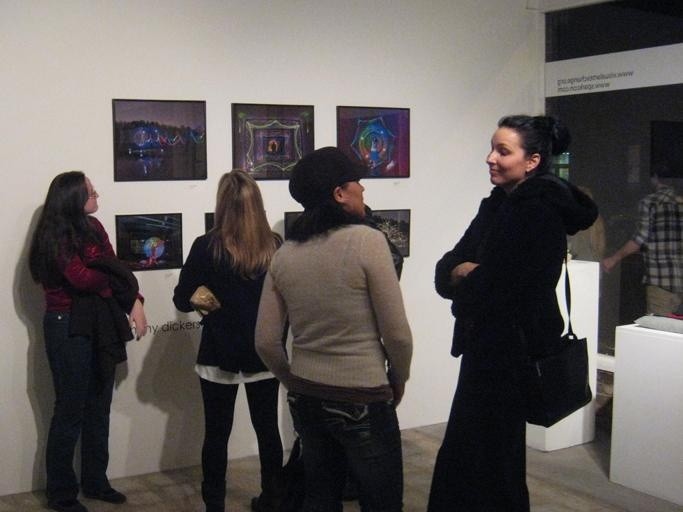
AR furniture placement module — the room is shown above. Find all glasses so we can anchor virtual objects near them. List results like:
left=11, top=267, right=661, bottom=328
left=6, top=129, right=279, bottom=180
left=85, top=189, right=97, bottom=200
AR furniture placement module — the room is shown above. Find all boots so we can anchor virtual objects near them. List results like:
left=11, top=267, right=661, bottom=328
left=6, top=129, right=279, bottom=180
left=201, top=479, right=226, bottom=512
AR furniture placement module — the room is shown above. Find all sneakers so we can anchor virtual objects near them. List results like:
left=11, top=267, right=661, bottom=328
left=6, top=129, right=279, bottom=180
left=82, top=487, right=127, bottom=504
left=251, top=497, right=260, bottom=511
left=47, top=497, right=88, bottom=511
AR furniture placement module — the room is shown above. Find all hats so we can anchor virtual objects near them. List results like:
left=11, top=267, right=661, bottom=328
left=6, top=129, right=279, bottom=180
left=288, top=146, right=368, bottom=209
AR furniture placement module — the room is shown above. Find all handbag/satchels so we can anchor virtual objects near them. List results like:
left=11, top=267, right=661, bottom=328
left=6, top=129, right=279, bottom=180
left=518, top=338, right=592, bottom=428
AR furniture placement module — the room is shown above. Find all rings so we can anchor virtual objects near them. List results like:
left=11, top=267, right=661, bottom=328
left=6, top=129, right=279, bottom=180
left=144, top=323, right=148, bottom=328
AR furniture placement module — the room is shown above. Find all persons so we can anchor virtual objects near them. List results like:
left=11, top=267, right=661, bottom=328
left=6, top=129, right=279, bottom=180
left=170, top=169, right=289, bottom=512
left=250, top=199, right=404, bottom=511
left=24, top=169, right=149, bottom=510
left=252, top=145, right=415, bottom=512
left=423, top=112, right=603, bottom=510
left=598, top=156, right=682, bottom=318
